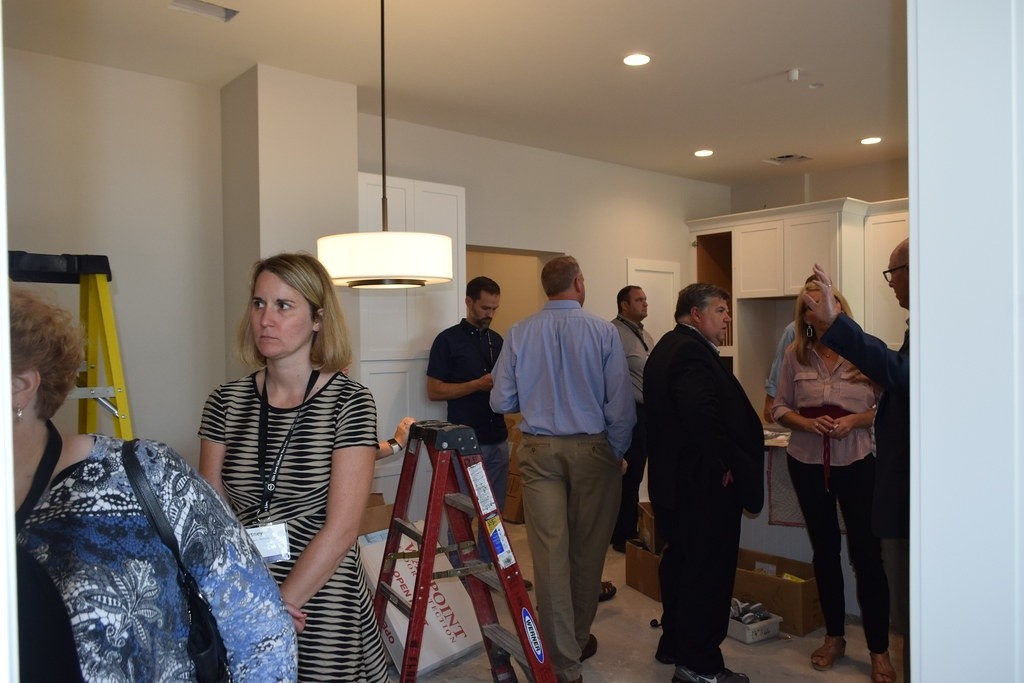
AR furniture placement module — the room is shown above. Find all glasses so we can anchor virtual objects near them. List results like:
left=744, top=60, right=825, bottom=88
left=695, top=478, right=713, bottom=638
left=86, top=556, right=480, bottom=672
left=883, top=265, right=906, bottom=283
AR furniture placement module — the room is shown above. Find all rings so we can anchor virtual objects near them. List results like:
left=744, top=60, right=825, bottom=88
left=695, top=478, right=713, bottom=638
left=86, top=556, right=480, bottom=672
left=815, top=423, right=820, bottom=428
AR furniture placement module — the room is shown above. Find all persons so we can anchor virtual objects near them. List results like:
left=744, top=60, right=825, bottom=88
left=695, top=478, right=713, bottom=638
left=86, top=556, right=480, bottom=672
left=642, top=282, right=766, bottom=683
left=763, top=272, right=819, bottom=424
left=598, top=581, right=617, bottom=602
left=607, top=287, right=655, bottom=553
left=801, top=236, right=910, bottom=675
left=198, top=253, right=392, bottom=683
left=426, top=277, right=533, bottom=594
left=769, top=282, right=896, bottom=683
left=488, top=255, right=637, bottom=683
left=10, top=282, right=300, bottom=683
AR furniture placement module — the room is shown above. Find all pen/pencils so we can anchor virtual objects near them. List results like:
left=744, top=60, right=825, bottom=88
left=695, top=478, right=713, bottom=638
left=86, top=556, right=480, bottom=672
left=484, top=369, right=489, bottom=374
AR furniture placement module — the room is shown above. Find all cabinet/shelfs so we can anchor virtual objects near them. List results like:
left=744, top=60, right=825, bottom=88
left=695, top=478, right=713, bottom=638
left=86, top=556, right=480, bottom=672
left=358, top=171, right=466, bottom=495
left=686, top=197, right=907, bottom=560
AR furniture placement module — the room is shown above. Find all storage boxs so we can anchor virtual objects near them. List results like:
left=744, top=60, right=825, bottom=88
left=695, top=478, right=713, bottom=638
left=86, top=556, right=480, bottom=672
left=733, top=550, right=826, bottom=636
left=356, top=521, right=486, bottom=677
left=502, top=475, right=524, bottom=523
left=625, top=538, right=665, bottom=602
left=727, top=611, right=784, bottom=644
left=638, top=502, right=661, bottom=553
left=354, top=503, right=409, bottom=539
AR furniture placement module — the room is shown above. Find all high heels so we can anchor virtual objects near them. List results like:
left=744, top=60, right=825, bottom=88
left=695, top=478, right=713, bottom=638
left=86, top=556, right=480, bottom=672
left=870, top=652, right=896, bottom=683
left=811, top=637, right=846, bottom=670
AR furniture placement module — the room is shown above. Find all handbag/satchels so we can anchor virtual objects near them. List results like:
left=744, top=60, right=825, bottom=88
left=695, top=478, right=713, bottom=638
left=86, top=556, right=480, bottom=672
left=122, top=439, right=233, bottom=683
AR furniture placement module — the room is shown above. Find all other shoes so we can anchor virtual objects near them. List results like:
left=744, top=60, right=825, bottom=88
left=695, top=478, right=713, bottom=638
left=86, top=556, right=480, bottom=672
left=612, top=543, right=627, bottom=553
left=599, top=582, right=616, bottom=602
left=523, top=579, right=533, bottom=590
left=580, top=634, right=597, bottom=664
left=570, top=675, right=582, bottom=683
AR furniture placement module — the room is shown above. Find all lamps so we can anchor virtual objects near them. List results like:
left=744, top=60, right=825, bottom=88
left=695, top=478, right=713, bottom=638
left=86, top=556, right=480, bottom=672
left=317, top=0, right=453, bottom=290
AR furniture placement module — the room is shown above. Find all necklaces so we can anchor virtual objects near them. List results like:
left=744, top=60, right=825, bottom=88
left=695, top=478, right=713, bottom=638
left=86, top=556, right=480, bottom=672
left=814, top=343, right=834, bottom=358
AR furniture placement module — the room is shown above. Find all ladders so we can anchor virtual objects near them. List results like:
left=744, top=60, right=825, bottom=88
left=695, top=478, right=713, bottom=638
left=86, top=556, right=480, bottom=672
left=7, top=250, right=134, bottom=441
left=375, top=420, right=558, bottom=683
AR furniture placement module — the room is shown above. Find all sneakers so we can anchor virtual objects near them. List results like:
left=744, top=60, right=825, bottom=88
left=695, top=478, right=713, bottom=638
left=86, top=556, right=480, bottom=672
left=655, top=650, right=676, bottom=664
left=672, top=665, right=750, bottom=683
